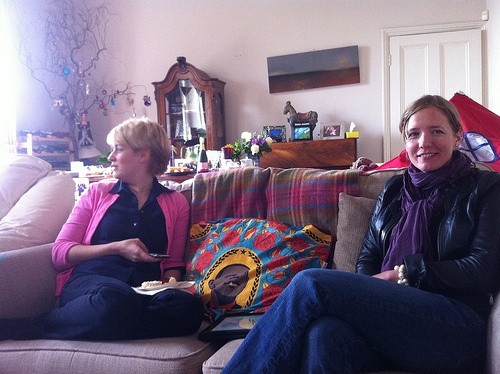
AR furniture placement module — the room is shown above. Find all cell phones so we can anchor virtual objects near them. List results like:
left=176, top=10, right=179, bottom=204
left=148, top=253, right=170, bottom=258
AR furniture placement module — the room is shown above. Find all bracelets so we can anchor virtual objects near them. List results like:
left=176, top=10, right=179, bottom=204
left=394, top=265, right=409, bottom=286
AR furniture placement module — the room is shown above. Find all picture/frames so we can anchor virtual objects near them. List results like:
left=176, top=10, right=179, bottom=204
left=320, top=121, right=345, bottom=140
left=263, top=125, right=287, bottom=143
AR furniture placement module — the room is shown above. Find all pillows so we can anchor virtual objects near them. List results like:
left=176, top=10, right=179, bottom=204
left=183, top=217, right=333, bottom=315
left=332, top=193, right=377, bottom=274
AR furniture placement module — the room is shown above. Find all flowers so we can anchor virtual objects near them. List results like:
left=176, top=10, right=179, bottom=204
left=224, top=131, right=275, bottom=164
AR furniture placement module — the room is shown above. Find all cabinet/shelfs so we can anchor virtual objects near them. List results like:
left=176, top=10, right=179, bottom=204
left=151, top=56, right=226, bottom=159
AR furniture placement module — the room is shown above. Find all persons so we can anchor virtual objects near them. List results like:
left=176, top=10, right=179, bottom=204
left=219, top=94, right=500, bottom=374
left=7, top=124, right=205, bottom=339
left=77, top=112, right=93, bottom=145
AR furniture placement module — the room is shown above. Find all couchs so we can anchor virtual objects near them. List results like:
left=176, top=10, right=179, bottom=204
left=0, top=152, right=500, bottom=374
